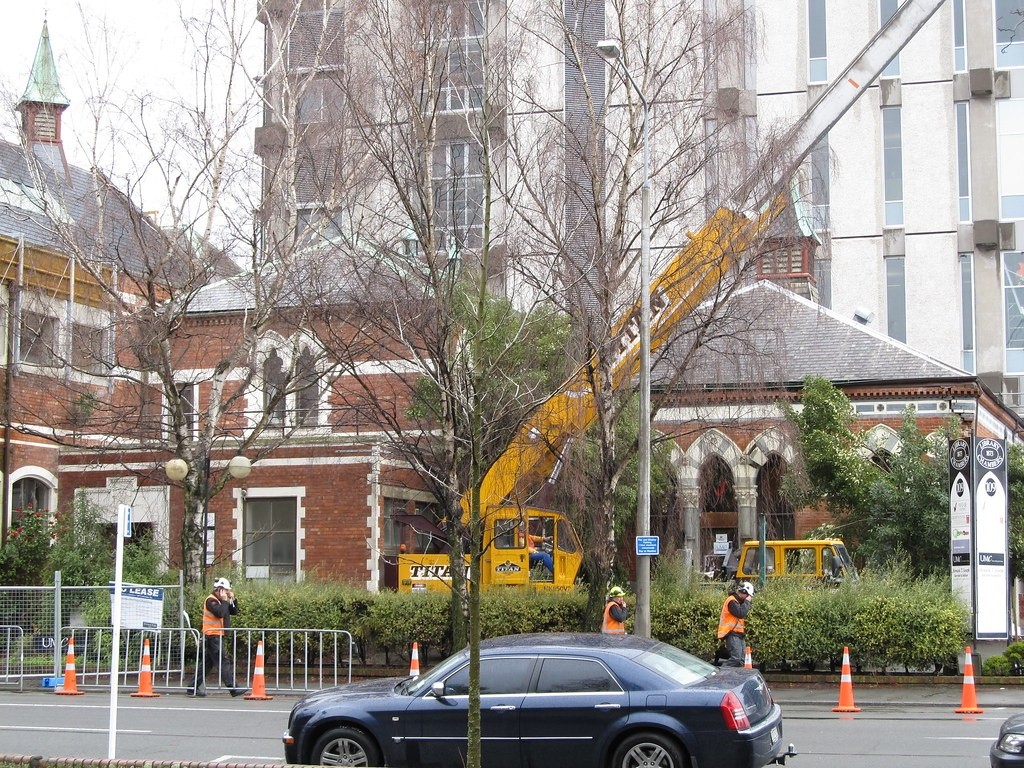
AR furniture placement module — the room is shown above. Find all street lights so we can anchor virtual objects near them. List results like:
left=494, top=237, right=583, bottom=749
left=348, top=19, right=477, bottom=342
left=596, top=39, right=652, bottom=637
left=162, top=437, right=251, bottom=689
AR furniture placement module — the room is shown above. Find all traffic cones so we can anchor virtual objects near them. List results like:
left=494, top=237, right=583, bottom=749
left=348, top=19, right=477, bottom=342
left=55, top=638, right=84, bottom=693
left=131, top=639, right=160, bottom=694
left=951, top=646, right=986, bottom=713
left=244, top=641, right=278, bottom=699
left=744, top=643, right=756, bottom=664
left=409, top=640, right=423, bottom=675
left=831, top=643, right=862, bottom=710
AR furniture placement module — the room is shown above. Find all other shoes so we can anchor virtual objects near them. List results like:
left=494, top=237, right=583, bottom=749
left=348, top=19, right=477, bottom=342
left=230, top=687, right=249, bottom=697
left=186, top=689, right=205, bottom=696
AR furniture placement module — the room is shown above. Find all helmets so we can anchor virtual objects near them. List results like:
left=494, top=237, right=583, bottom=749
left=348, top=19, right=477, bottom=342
left=608, top=586, right=626, bottom=597
left=214, top=577, right=232, bottom=590
left=739, top=581, right=754, bottom=596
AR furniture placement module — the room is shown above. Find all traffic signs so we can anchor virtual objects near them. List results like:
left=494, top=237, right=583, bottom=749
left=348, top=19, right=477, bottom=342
left=632, top=529, right=666, bottom=565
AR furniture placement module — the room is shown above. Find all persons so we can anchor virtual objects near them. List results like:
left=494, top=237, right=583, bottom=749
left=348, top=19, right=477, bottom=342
left=186, top=577, right=248, bottom=697
left=510, top=521, right=553, bottom=573
left=717, top=582, right=755, bottom=667
left=602, top=586, right=627, bottom=634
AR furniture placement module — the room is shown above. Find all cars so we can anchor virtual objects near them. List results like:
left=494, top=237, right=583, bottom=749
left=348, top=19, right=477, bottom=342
left=712, top=531, right=861, bottom=587
left=281, top=636, right=797, bottom=768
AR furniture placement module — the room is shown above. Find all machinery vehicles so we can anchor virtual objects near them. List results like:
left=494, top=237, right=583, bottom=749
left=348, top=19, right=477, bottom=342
left=378, top=0, right=946, bottom=587
